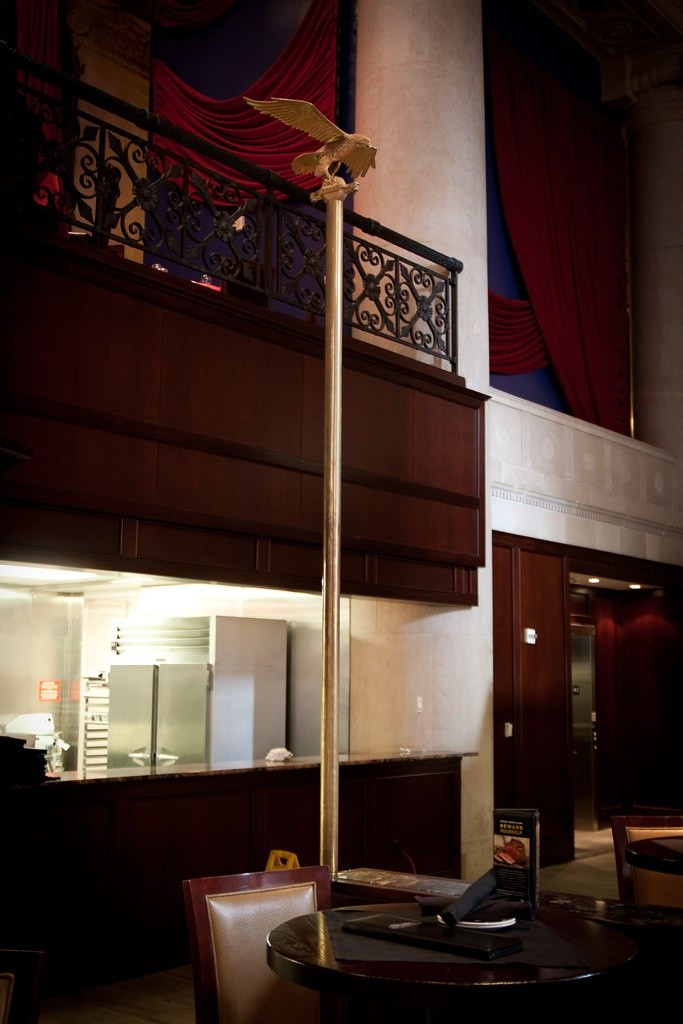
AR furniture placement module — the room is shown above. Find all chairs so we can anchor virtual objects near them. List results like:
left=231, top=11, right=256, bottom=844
left=610, top=813, right=683, bottom=906
left=182, top=864, right=332, bottom=1024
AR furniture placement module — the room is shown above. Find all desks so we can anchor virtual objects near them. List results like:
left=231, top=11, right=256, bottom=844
left=268, top=904, right=638, bottom=1024
left=626, top=836, right=683, bottom=877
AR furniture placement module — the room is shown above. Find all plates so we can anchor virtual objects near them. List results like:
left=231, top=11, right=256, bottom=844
left=437, top=915, right=516, bottom=928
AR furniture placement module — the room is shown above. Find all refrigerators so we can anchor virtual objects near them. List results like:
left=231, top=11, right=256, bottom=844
left=106, top=665, right=207, bottom=770
